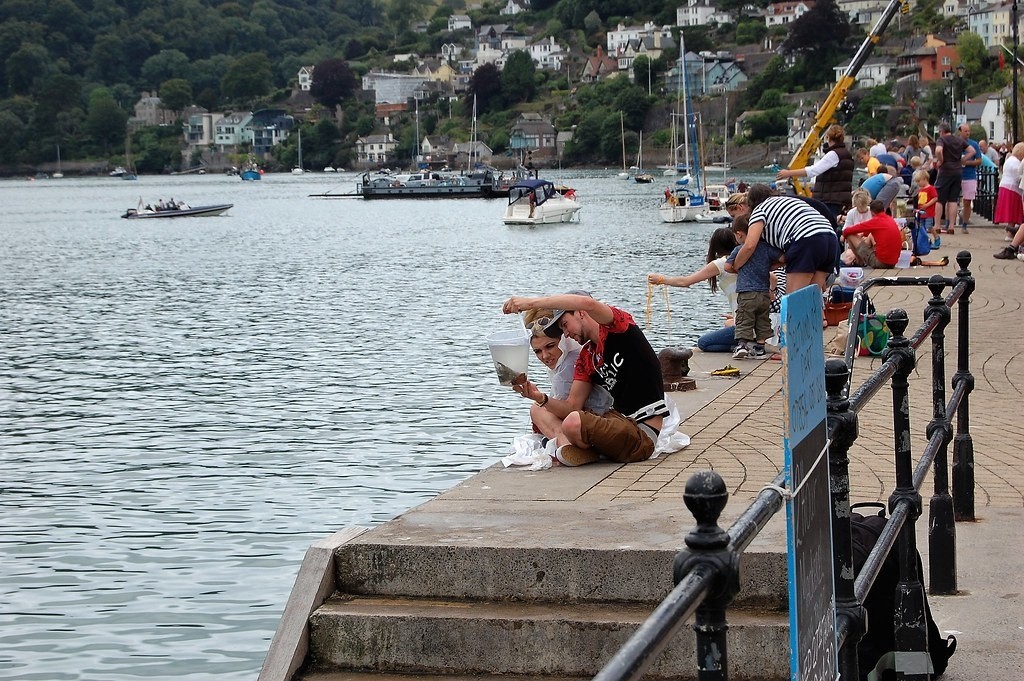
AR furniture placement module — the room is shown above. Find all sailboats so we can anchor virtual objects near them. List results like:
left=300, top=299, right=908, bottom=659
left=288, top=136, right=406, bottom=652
left=634, top=130, right=655, bottom=184
left=658, top=29, right=740, bottom=224
left=618, top=109, right=629, bottom=179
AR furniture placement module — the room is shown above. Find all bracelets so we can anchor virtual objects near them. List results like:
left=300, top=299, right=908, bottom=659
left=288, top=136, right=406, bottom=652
left=769, top=287, right=779, bottom=296
left=659, top=275, right=665, bottom=284
left=535, top=393, right=548, bottom=407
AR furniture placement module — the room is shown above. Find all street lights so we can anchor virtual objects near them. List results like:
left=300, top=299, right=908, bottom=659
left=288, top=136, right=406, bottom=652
left=943, top=65, right=966, bottom=134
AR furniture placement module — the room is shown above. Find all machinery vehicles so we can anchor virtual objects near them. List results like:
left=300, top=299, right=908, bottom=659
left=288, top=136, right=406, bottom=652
left=769, top=0, right=904, bottom=198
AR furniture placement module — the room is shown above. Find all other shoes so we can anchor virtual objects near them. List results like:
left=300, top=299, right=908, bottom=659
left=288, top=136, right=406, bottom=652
left=947, top=230, right=955, bottom=234
left=555, top=444, right=600, bottom=466
left=936, top=228, right=943, bottom=234
left=963, top=228, right=968, bottom=234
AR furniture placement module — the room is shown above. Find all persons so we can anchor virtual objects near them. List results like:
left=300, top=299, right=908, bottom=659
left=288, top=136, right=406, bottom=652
left=775, top=125, right=854, bottom=217
left=523, top=308, right=613, bottom=435
left=732, top=183, right=838, bottom=360
left=720, top=265, right=787, bottom=328
left=724, top=191, right=839, bottom=232
left=842, top=200, right=903, bottom=269
left=648, top=227, right=739, bottom=352
left=857, top=123, right=1024, bottom=260
left=840, top=187, right=873, bottom=251
left=502, top=290, right=670, bottom=467
left=723, top=213, right=786, bottom=360
left=528, top=190, right=535, bottom=218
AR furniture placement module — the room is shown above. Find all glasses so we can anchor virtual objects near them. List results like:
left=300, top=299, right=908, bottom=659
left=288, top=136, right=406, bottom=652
left=526, top=317, right=550, bottom=330
left=724, top=201, right=742, bottom=208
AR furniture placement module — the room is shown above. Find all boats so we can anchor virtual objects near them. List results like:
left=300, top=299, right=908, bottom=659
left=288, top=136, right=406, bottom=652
left=120, top=203, right=235, bottom=220
left=361, top=162, right=579, bottom=198
left=239, top=151, right=261, bottom=180
left=110, top=166, right=128, bottom=176
left=501, top=179, right=584, bottom=225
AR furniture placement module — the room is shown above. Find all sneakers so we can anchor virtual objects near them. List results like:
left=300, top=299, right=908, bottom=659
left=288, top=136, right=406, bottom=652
left=733, top=345, right=748, bottom=360
left=994, top=246, right=1015, bottom=259
left=749, top=348, right=767, bottom=360
left=934, top=236, right=940, bottom=247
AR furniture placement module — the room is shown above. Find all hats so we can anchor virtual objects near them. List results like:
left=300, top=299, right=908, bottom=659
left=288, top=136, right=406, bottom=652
left=935, top=123, right=950, bottom=130
left=543, top=289, right=593, bottom=338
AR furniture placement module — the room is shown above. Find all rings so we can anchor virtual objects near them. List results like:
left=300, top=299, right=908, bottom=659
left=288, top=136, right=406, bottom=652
left=521, top=391, right=523, bottom=396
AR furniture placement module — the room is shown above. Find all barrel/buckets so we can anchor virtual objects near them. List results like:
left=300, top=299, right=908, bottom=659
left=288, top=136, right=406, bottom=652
left=895, top=241, right=913, bottom=268
left=840, top=267, right=863, bottom=289
left=764, top=311, right=780, bottom=348
left=487, top=312, right=533, bottom=387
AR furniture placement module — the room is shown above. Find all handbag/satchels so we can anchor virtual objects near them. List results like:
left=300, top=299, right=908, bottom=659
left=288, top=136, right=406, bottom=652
left=825, top=286, right=890, bottom=358
left=912, top=210, right=931, bottom=255
